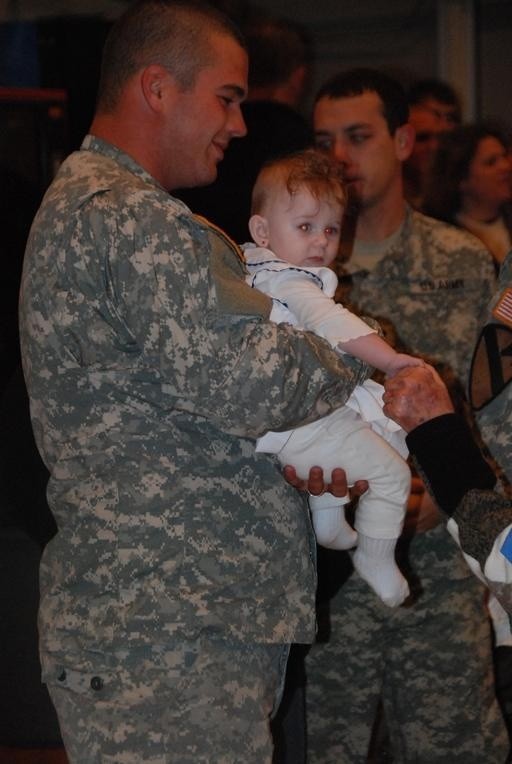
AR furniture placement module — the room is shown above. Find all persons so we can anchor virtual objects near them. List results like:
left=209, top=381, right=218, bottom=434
left=2, top=0, right=511, bottom=764
